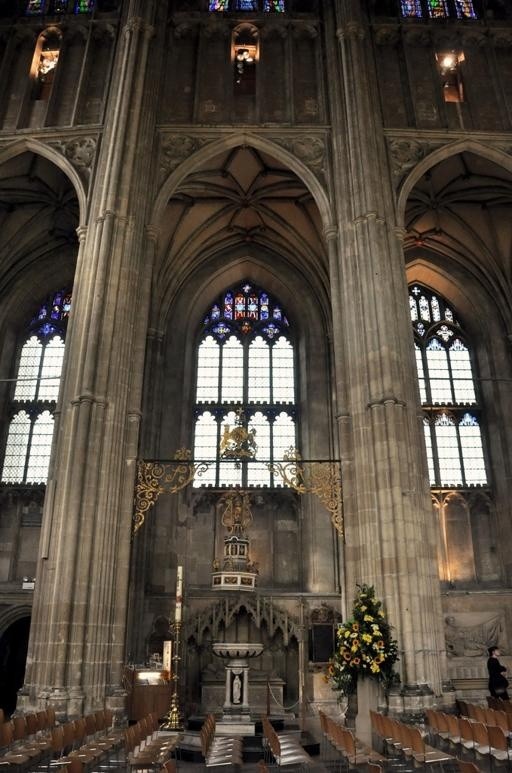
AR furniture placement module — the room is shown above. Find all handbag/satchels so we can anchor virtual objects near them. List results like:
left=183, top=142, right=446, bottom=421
left=494, top=688, right=505, bottom=695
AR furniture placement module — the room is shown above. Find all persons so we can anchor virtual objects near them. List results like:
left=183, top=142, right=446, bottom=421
left=483, top=643, right=508, bottom=699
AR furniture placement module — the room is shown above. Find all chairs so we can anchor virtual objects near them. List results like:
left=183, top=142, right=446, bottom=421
left=0, top=697, right=512, bottom=773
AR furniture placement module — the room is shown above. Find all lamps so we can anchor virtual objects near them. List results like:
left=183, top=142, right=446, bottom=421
left=22, top=576, right=36, bottom=590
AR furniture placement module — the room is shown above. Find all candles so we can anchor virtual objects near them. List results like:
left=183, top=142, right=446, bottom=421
left=175, top=565, right=183, bottom=621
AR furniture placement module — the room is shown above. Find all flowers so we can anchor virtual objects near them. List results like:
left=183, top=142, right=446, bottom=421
left=321, top=582, right=401, bottom=703
